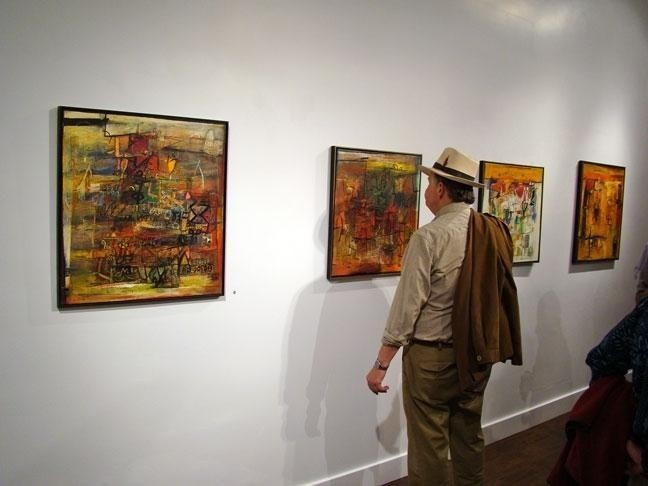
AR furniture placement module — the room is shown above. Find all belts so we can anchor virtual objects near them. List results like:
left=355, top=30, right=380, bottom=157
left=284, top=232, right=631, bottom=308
left=408, top=336, right=456, bottom=351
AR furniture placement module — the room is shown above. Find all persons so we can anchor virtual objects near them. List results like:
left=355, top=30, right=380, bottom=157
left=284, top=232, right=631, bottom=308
left=367, top=147, right=523, bottom=486
left=633, top=244, right=646, bottom=308
left=548, top=295, right=647, bottom=484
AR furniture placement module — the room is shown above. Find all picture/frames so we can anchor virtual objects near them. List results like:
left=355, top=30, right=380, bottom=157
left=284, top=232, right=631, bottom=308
left=325, top=145, right=422, bottom=280
left=477, top=160, right=545, bottom=267
left=573, top=159, right=625, bottom=264
left=57, top=105, right=227, bottom=312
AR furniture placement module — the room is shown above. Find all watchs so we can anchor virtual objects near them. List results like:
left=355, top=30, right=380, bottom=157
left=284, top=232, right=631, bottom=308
left=374, top=359, right=390, bottom=370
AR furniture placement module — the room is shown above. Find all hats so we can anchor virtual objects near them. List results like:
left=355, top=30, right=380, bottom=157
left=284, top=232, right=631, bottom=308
left=417, top=145, right=486, bottom=192
